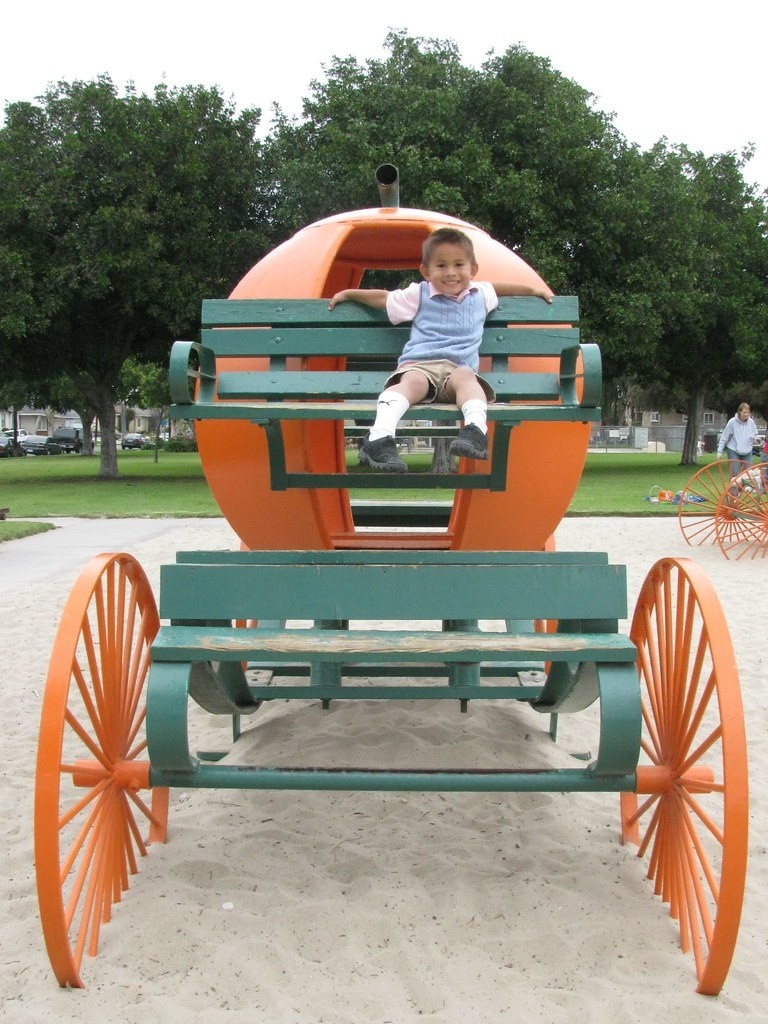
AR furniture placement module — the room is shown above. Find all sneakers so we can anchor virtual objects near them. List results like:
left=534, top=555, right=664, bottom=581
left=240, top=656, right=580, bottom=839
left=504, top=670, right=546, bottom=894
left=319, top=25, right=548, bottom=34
left=357, top=432, right=408, bottom=473
left=449, top=421, right=490, bottom=461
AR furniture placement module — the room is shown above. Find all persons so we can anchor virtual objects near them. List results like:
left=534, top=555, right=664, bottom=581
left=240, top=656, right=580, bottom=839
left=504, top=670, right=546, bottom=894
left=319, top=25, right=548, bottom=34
left=717, top=403, right=758, bottom=503
left=328, top=227, right=554, bottom=472
left=758, top=436, right=768, bottom=489
left=696, top=436, right=704, bottom=457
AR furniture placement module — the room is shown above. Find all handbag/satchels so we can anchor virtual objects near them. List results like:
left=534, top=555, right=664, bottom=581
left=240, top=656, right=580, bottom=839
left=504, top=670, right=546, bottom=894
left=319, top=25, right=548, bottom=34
left=659, top=490, right=672, bottom=501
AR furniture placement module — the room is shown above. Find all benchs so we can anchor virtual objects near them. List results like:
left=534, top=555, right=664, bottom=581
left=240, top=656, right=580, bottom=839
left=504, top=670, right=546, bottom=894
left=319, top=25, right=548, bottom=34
left=168, top=301, right=603, bottom=490
left=145, top=547, right=637, bottom=791
left=730, top=452, right=768, bottom=521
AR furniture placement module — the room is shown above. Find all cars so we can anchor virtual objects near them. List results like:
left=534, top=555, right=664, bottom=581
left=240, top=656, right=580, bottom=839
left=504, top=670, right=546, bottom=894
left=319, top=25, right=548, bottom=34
left=115, top=429, right=125, bottom=441
left=121, top=433, right=149, bottom=450
left=160, top=432, right=170, bottom=442
left=0, top=428, right=53, bottom=458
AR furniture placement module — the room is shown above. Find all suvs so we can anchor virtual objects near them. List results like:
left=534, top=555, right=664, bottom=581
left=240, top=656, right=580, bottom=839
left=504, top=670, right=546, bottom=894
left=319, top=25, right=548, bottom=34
left=43, top=425, right=97, bottom=455
